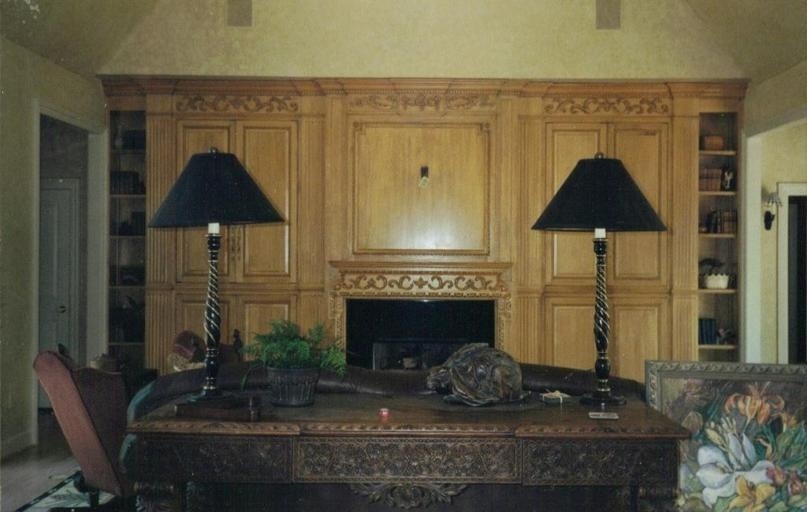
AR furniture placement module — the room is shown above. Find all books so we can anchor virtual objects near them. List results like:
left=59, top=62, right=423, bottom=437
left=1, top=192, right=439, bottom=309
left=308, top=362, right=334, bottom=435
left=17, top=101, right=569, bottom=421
left=699, top=166, right=737, bottom=234
left=699, top=317, right=717, bottom=345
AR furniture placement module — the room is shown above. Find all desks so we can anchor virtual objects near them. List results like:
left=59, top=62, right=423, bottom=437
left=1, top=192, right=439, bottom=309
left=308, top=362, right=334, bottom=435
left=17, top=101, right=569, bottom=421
left=126, top=394, right=692, bottom=512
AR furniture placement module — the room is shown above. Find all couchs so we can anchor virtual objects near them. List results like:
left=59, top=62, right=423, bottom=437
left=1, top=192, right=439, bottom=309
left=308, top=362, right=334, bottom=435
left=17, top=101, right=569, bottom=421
left=126, top=366, right=669, bottom=511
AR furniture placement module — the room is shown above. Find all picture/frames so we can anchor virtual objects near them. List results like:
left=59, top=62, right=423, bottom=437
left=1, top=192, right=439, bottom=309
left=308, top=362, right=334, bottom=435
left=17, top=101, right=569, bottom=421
left=642, top=361, right=807, bottom=512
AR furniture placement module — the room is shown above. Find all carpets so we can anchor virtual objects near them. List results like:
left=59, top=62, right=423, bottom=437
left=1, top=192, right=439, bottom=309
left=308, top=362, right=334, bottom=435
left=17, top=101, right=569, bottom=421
left=16, top=469, right=131, bottom=511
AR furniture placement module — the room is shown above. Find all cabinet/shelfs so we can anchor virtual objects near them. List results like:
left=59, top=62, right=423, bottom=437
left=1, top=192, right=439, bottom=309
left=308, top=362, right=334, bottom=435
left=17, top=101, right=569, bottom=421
left=535, top=78, right=749, bottom=386
left=101, top=75, right=324, bottom=378
left=324, top=81, right=534, bottom=372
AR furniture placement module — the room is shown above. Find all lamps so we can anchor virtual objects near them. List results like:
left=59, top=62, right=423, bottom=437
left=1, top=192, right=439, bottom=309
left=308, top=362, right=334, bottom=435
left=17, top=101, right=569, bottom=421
left=529, top=153, right=667, bottom=409
left=764, top=193, right=783, bottom=229
left=146, top=147, right=288, bottom=410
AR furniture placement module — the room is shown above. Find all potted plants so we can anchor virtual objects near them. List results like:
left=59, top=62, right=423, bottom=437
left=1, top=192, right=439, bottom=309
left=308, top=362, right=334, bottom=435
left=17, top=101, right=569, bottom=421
left=699, top=257, right=731, bottom=289
left=244, top=322, right=344, bottom=406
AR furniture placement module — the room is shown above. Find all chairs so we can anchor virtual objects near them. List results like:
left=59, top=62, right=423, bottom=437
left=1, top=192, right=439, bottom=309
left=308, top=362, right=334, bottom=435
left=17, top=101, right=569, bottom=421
left=32, top=346, right=132, bottom=510
